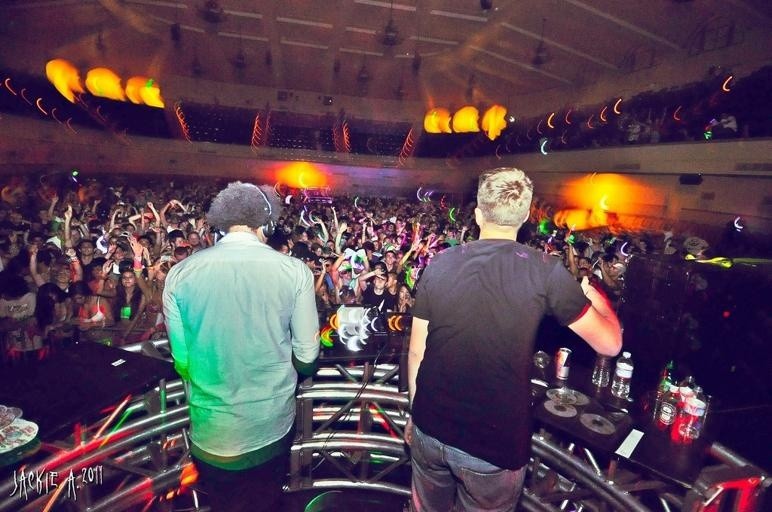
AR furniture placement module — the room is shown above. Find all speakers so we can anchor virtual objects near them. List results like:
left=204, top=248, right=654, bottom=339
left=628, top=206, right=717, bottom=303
left=680, top=175, right=703, bottom=185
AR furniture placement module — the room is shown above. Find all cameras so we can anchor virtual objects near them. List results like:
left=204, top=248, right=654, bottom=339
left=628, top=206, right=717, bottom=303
left=592, top=256, right=600, bottom=264
left=161, top=255, right=173, bottom=262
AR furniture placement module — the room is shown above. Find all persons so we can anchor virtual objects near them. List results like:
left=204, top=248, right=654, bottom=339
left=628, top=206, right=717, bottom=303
left=405, top=167, right=623, bottom=511
left=534, top=79, right=737, bottom=150
left=0, top=172, right=652, bottom=345
left=161, top=180, right=319, bottom=512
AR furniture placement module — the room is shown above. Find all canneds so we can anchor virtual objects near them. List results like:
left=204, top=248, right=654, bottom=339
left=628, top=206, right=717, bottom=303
left=556, top=347, right=573, bottom=381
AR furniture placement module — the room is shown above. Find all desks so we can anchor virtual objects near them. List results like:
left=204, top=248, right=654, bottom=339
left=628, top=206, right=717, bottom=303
left=0, top=307, right=772, bottom=512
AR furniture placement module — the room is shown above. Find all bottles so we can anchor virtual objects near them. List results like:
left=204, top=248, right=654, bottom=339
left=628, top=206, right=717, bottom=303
left=591, top=352, right=611, bottom=388
left=610, top=351, right=634, bottom=400
left=651, top=360, right=712, bottom=447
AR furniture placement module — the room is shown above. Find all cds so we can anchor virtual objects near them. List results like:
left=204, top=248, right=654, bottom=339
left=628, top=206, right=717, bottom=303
left=546, top=386, right=589, bottom=406
left=579, top=413, right=617, bottom=436
left=544, top=400, right=577, bottom=418
